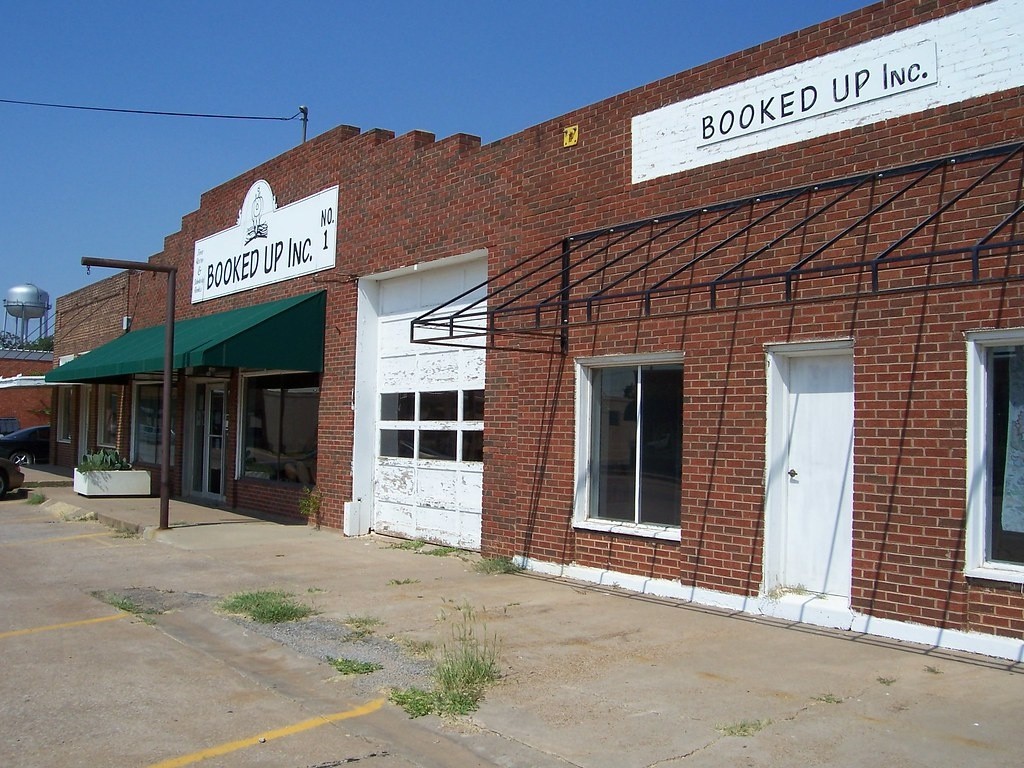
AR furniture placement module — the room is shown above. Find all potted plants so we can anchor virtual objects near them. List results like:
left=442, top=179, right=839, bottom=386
left=73, top=446, right=151, bottom=496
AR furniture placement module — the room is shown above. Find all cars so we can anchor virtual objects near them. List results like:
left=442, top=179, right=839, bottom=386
left=0, top=426, right=50, bottom=467
left=0, top=457, right=24, bottom=500
